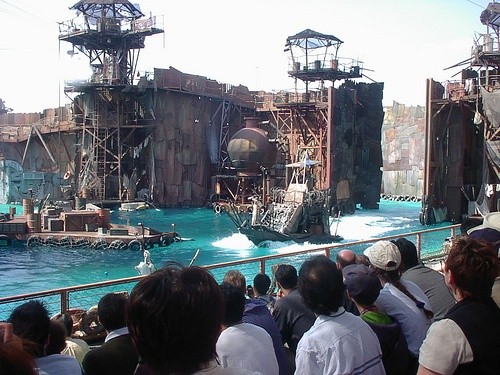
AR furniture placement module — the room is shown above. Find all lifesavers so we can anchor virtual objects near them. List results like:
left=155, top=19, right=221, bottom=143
left=91, top=239, right=109, bottom=250
left=128, top=240, right=142, bottom=252
left=44, top=236, right=60, bottom=247
left=109, top=240, right=128, bottom=250
left=145, top=238, right=154, bottom=250
left=27, top=236, right=44, bottom=247
left=60, top=237, right=75, bottom=249
left=75, top=239, right=91, bottom=249
left=158, top=236, right=170, bottom=247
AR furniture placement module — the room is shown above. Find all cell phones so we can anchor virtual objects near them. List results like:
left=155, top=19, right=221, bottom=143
left=247, top=285, right=254, bottom=294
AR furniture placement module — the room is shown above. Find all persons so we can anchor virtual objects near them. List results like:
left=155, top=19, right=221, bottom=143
left=292, top=256, right=386, bottom=375
left=1, top=263, right=316, bottom=375
left=336, top=212, right=500, bottom=375
left=248, top=195, right=264, bottom=226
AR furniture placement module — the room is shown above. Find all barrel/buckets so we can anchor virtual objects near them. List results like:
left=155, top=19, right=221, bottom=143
left=75, top=198, right=86, bottom=210
left=27, top=214, right=41, bottom=233
left=22, top=198, right=31, bottom=216
left=98, top=209, right=110, bottom=229
left=9, top=207, right=16, bottom=219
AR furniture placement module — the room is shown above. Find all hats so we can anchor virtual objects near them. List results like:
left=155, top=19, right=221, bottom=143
left=390, top=238, right=417, bottom=256
left=467, top=212, right=500, bottom=236
left=363, top=240, right=401, bottom=271
left=468, top=228, right=500, bottom=249
left=342, top=264, right=381, bottom=296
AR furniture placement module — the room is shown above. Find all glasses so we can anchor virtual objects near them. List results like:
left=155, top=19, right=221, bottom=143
left=440, top=259, right=456, bottom=273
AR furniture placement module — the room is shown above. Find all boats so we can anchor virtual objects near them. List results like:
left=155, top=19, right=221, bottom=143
left=225, top=176, right=345, bottom=249
left=0, top=185, right=196, bottom=251
